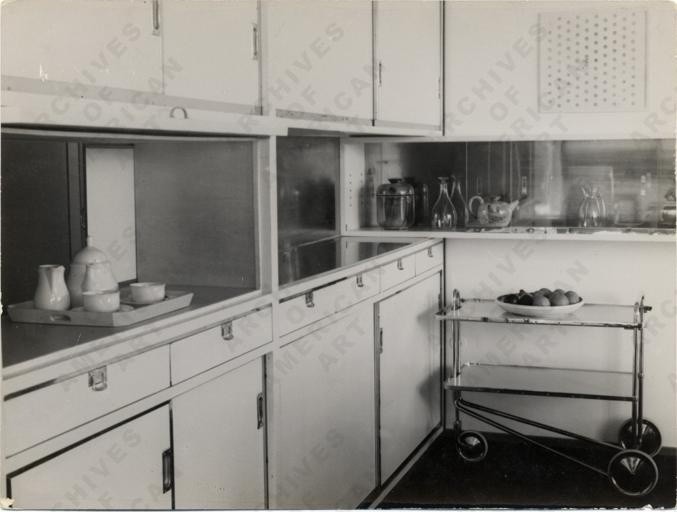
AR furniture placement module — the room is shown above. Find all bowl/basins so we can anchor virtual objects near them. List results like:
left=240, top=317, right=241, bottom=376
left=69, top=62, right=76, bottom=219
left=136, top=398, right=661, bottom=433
left=129, top=280, right=166, bottom=304
left=84, top=290, right=119, bottom=313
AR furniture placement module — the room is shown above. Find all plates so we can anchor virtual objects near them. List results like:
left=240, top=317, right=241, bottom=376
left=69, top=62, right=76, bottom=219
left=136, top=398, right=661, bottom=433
left=496, top=294, right=582, bottom=314
left=69, top=305, right=134, bottom=319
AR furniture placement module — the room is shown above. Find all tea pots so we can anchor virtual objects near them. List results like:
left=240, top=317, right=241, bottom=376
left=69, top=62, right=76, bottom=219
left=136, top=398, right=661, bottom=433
left=66, top=237, right=120, bottom=305
left=32, top=265, right=71, bottom=310
left=468, top=194, right=519, bottom=228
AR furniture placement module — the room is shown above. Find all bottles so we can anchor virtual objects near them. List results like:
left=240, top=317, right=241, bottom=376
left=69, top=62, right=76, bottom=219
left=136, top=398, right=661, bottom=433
left=431, top=174, right=457, bottom=228
left=576, top=185, right=600, bottom=231
left=448, top=176, right=469, bottom=226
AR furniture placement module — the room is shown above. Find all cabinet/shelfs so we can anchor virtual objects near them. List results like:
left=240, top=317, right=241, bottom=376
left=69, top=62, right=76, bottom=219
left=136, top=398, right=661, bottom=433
left=0, top=240, right=446, bottom=509
left=1, top=0, right=165, bottom=100
left=164, top=1, right=261, bottom=110
left=374, top=0, right=444, bottom=129
left=261, top=0, right=373, bottom=125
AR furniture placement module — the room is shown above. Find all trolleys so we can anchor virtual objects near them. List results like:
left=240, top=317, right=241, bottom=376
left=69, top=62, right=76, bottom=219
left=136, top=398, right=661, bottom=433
left=435, top=288, right=662, bottom=497
